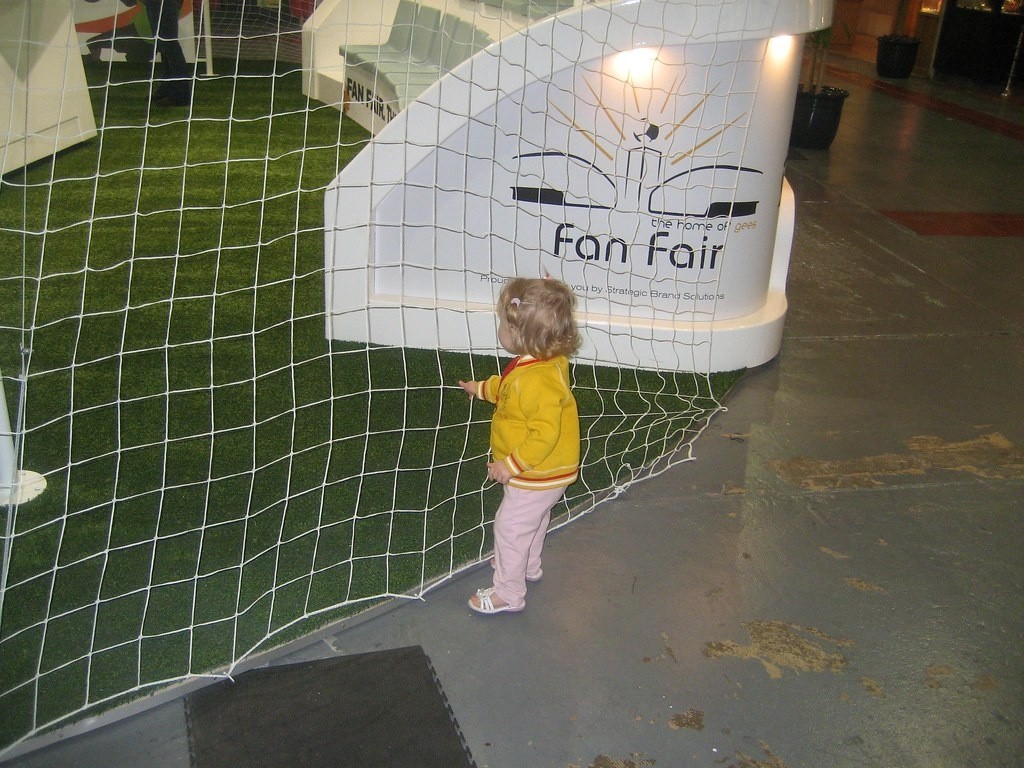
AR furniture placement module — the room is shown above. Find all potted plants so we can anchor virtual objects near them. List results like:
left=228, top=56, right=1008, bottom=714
left=789, top=20, right=852, bottom=150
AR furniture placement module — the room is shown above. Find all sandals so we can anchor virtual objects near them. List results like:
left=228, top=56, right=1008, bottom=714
left=468, top=586, right=525, bottom=615
left=490, top=559, right=544, bottom=582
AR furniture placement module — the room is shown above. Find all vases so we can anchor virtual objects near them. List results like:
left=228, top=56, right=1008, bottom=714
left=874, top=33, right=922, bottom=79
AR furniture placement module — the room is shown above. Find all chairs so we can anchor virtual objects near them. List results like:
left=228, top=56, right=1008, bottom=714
left=340, top=0, right=574, bottom=112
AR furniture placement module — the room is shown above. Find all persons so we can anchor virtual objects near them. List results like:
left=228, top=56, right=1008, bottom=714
left=458, top=277, right=584, bottom=615
left=144, top=0, right=192, bottom=109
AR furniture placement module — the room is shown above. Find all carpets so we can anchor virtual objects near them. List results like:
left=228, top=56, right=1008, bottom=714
left=183, top=644, right=479, bottom=768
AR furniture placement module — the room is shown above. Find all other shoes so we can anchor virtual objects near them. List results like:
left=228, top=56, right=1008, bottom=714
left=144, top=86, right=175, bottom=105
left=167, top=93, right=188, bottom=108
